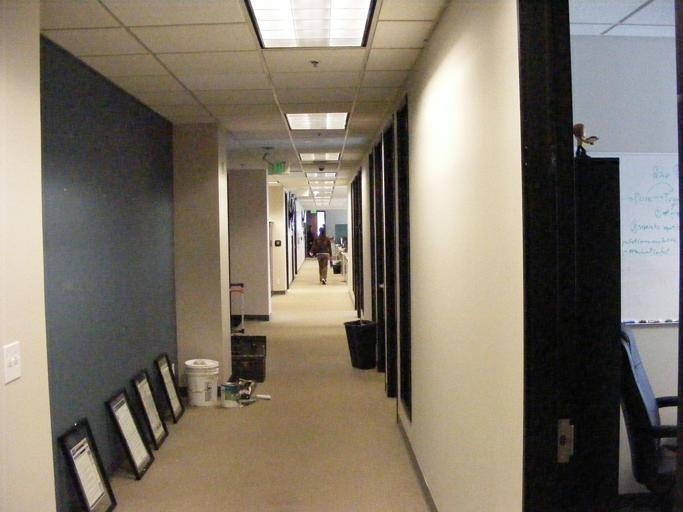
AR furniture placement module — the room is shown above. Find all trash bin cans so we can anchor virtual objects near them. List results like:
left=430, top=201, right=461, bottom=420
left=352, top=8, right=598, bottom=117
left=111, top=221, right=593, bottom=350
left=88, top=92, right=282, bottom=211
left=332, top=265, right=340, bottom=274
left=343, top=317, right=377, bottom=370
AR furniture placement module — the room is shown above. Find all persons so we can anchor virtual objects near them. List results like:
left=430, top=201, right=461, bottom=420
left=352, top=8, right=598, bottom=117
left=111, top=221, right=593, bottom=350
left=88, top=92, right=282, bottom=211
left=309, top=228, right=332, bottom=284
left=307, top=222, right=325, bottom=257
left=573, top=124, right=598, bottom=158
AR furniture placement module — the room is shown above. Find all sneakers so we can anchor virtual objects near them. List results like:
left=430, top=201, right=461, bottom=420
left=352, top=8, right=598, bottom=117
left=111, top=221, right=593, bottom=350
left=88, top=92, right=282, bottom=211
left=322, top=279, right=326, bottom=284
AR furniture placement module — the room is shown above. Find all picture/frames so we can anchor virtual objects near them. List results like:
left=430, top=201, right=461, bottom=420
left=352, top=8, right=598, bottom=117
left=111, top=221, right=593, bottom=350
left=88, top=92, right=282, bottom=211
left=57, top=352, right=185, bottom=511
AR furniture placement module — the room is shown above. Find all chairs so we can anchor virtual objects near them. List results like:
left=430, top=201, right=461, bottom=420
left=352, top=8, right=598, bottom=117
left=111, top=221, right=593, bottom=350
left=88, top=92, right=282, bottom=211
left=616, top=320, right=682, bottom=499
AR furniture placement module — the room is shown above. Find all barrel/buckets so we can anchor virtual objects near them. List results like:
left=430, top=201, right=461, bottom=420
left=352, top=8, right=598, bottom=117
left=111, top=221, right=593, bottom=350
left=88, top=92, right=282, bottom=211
left=220, top=381, right=241, bottom=409
left=184, top=359, right=220, bottom=406
